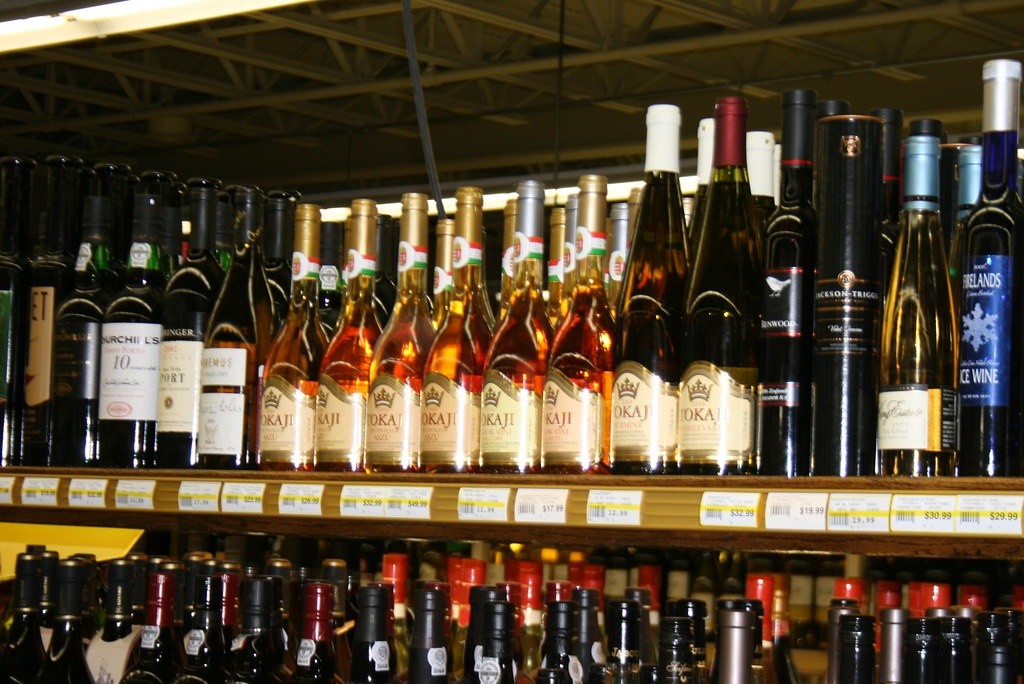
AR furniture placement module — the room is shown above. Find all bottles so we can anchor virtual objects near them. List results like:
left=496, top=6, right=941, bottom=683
left=0, top=56, right=1024, bottom=684
